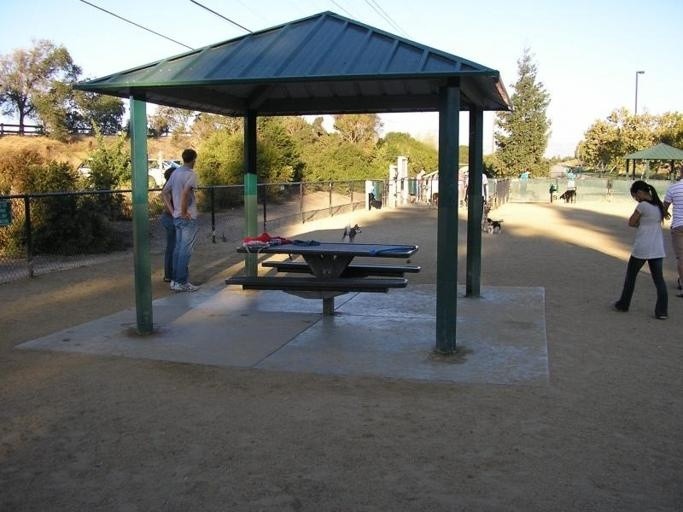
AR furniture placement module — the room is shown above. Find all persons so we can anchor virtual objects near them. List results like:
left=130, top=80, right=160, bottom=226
left=519, top=171, right=530, bottom=196
left=162, top=148, right=200, bottom=292
left=663, top=165, right=683, bottom=298
left=159, top=167, right=177, bottom=282
left=612, top=180, right=670, bottom=320
left=464, top=173, right=489, bottom=205
left=567, top=169, right=576, bottom=187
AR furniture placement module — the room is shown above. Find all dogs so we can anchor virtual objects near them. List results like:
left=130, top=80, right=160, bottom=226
left=559, top=187, right=576, bottom=203
left=342, top=224, right=361, bottom=243
left=480, top=205, right=491, bottom=231
left=486, top=218, right=503, bottom=234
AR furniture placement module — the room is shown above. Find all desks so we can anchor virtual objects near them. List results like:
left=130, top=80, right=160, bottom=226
left=237, top=240, right=419, bottom=316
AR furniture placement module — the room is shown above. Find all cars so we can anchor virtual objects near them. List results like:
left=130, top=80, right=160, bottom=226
left=79, top=158, right=181, bottom=190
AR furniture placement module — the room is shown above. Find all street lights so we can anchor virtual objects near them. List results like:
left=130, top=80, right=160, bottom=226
left=632, top=71, right=644, bottom=180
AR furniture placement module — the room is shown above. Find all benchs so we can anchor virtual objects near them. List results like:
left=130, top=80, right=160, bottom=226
left=226, top=275, right=408, bottom=300
left=261, top=260, right=420, bottom=277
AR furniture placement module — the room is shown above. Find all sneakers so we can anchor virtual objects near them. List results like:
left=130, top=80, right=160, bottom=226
left=612, top=304, right=627, bottom=312
left=656, top=313, right=667, bottom=318
left=165, top=279, right=201, bottom=292
